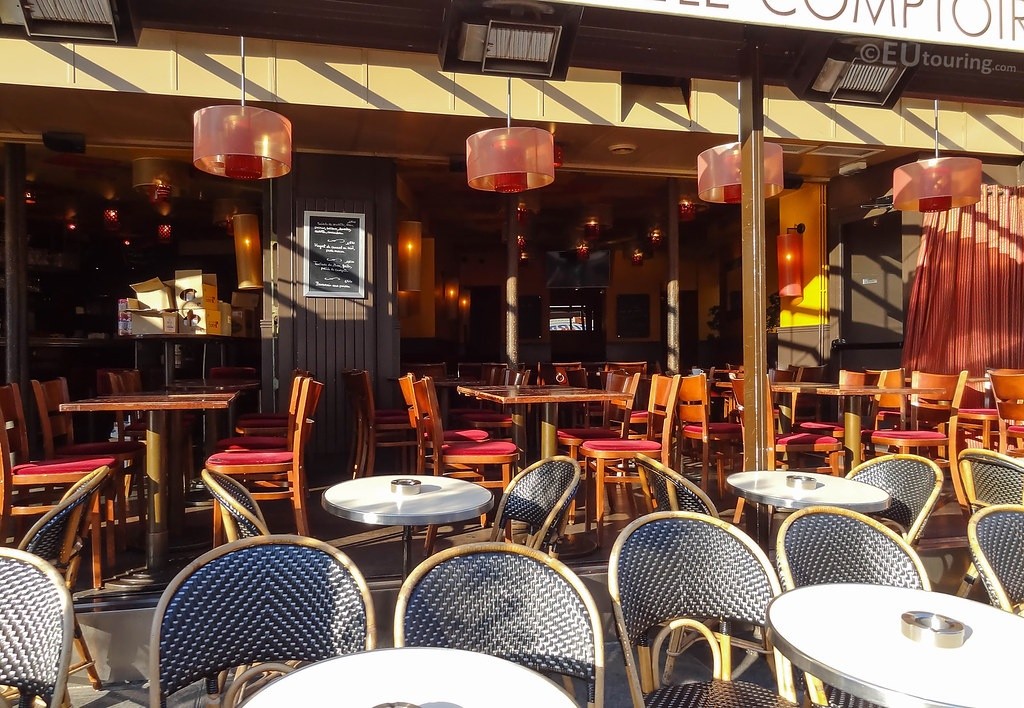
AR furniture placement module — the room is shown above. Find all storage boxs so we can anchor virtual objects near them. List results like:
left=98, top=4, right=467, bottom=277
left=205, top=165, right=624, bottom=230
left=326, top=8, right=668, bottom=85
left=125, top=270, right=260, bottom=338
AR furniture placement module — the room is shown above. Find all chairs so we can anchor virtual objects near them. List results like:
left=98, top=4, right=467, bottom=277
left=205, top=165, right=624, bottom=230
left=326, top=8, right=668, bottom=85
left=1, top=361, right=1024, bottom=708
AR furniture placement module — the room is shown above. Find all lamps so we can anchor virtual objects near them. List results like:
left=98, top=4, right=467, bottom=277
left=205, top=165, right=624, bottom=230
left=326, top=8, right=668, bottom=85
left=194, top=36, right=291, bottom=179
left=777, top=234, right=803, bottom=298
left=698, top=83, right=784, bottom=203
left=231, top=214, right=263, bottom=289
left=133, top=157, right=178, bottom=198
left=213, top=198, right=251, bottom=235
left=893, top=100, right=982, bottom=213
left=466, top=77, right=554, bottom=192
left=398, top=221, right=422, bottom=292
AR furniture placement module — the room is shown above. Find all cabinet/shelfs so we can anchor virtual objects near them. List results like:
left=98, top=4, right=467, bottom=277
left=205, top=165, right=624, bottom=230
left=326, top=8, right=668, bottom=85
left=0, top=215, right=127, bottom=342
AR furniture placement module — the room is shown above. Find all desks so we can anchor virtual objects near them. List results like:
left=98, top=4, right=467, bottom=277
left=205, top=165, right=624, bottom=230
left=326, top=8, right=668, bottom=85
left=430, top=377, right=486, bottom=429
left=124, top=333, right=226, bottom=387
left=475, top=390, right=633, bottom=557
left=58, top=397, right=237, bottom=594
left=96, top=391, right=241, bottom=551
left=765, top=582, right=1024, bottom=708
left=723, top=470, right=892, bottom=515
left=715, top=381, right=878, bottom=436
left=321, top=473, right=496, bottom=585
left=635, top=377, right=651, bottom=409
left=233, top=646, right=583, bottom=708
left=964, top=378, right=991, bottom=383
left=814, top=386, right=946, bottom=478
left=456, top=384, right=587, bottom=528
left=165, top=378, right=260, bottom=489
